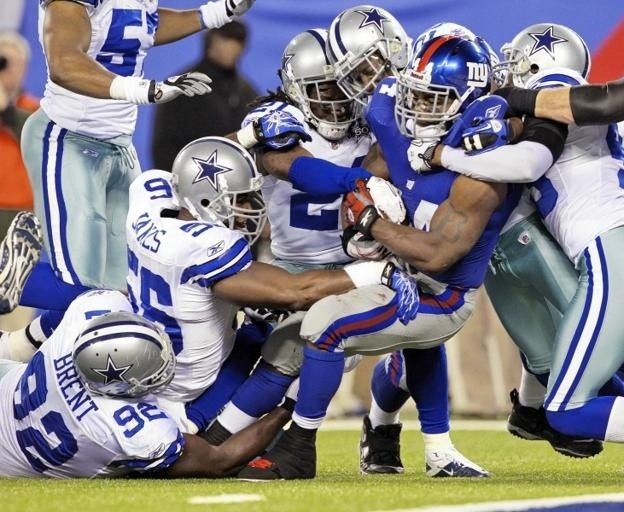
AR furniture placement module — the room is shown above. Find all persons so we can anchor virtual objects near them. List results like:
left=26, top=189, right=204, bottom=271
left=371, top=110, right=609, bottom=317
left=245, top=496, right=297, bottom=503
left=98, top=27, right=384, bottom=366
left=1, top=1, right=624, bottom=479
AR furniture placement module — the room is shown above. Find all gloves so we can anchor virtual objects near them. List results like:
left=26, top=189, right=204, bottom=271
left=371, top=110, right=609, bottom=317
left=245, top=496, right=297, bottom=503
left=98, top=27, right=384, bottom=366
left=494, top=86, right=540, bottom=119
left=337, top=225, right=392, bottom=261
left=200, top=0, right=255, bottom=30
left=340, top=176, right=380, bottom=240
left=341, top=354, right=364, bottom=373
left=110, top=70, right=214, bottom=105
left=363, top=174, right=409, bottom=227
left=380, top=262, right=421, bottom=326
left=252, top=110, right=313, bottom=151
left=403, top=138, right=441, bottom=174
left=459, top=116, right=514, bottom=158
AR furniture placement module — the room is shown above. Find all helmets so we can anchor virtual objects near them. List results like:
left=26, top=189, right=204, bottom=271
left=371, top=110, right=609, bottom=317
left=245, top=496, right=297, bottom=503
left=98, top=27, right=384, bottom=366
left=325, top=4, right=413, bottom=106
left=499, top=22, right=592, bottom=91
left=278, top=26, right=363, bottom=140
left=73, top=310, right=178, bottom=401
left=392, top=34, right=492, bottom=141
left=410, top=21, right=499, bottom=69
left=171, top=135, right=269, bottom=246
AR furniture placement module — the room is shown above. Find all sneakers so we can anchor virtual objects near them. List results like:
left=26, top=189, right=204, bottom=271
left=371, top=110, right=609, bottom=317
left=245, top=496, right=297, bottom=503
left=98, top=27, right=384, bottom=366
left=425, top=445, right=492, bottom=479
left=359, top=412, right=405, bottom=475
left=506, top=387, right=605, bottom=460
left=0, top=210, right=44, bottom=316
left=238, top=429, right=317, bottom=480
